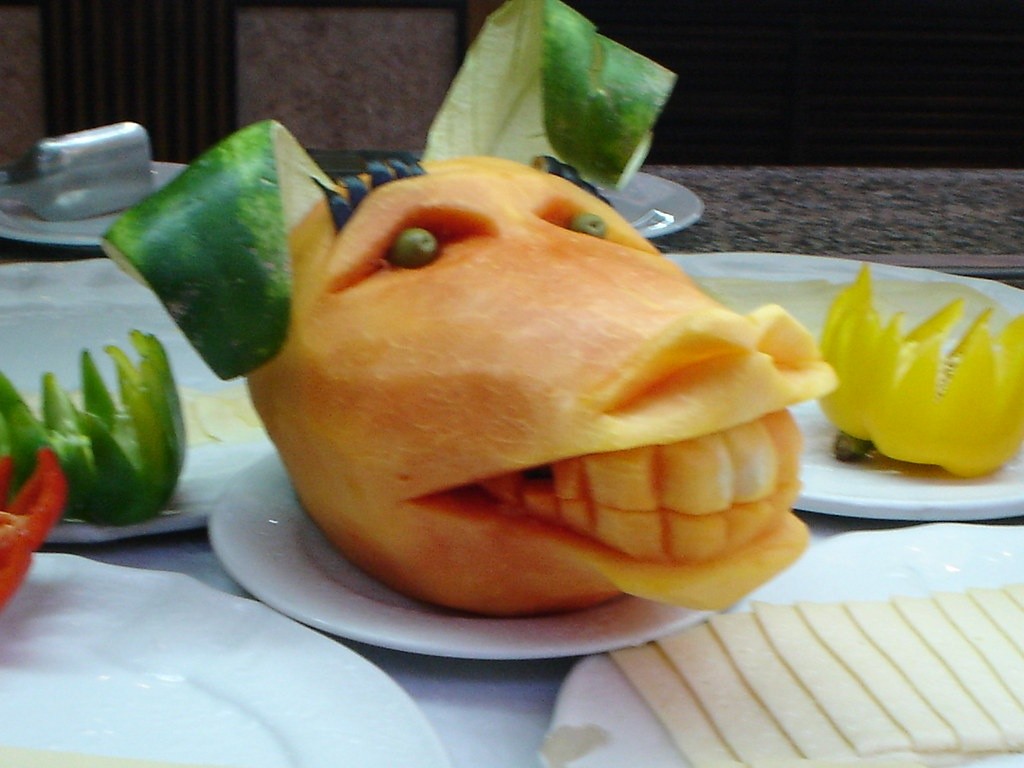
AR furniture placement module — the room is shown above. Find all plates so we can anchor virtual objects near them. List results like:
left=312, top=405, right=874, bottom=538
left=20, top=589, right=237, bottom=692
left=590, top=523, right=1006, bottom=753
left=0, top=554, right=450, bottom=762
left=576, top=172, right=703, bottom=238
left=211, top=446, right=735, bottom=661
left=661, top=252, right=1024, bottom=520
left=2, top=159, right=202, bottom=246
left=535, top=523, right=1024, bottom=766
left=2, top=260, right=279, bottom=539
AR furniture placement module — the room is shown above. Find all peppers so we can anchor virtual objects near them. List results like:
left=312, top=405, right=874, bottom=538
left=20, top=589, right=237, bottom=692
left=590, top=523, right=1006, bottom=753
left=822, top=265, right=1024, bottom=479
left=0, top=329, right=189, bottom=627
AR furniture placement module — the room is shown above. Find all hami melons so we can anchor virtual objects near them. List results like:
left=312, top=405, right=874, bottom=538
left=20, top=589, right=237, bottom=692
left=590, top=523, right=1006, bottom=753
left=249, top=150, right=840, bottom=618
